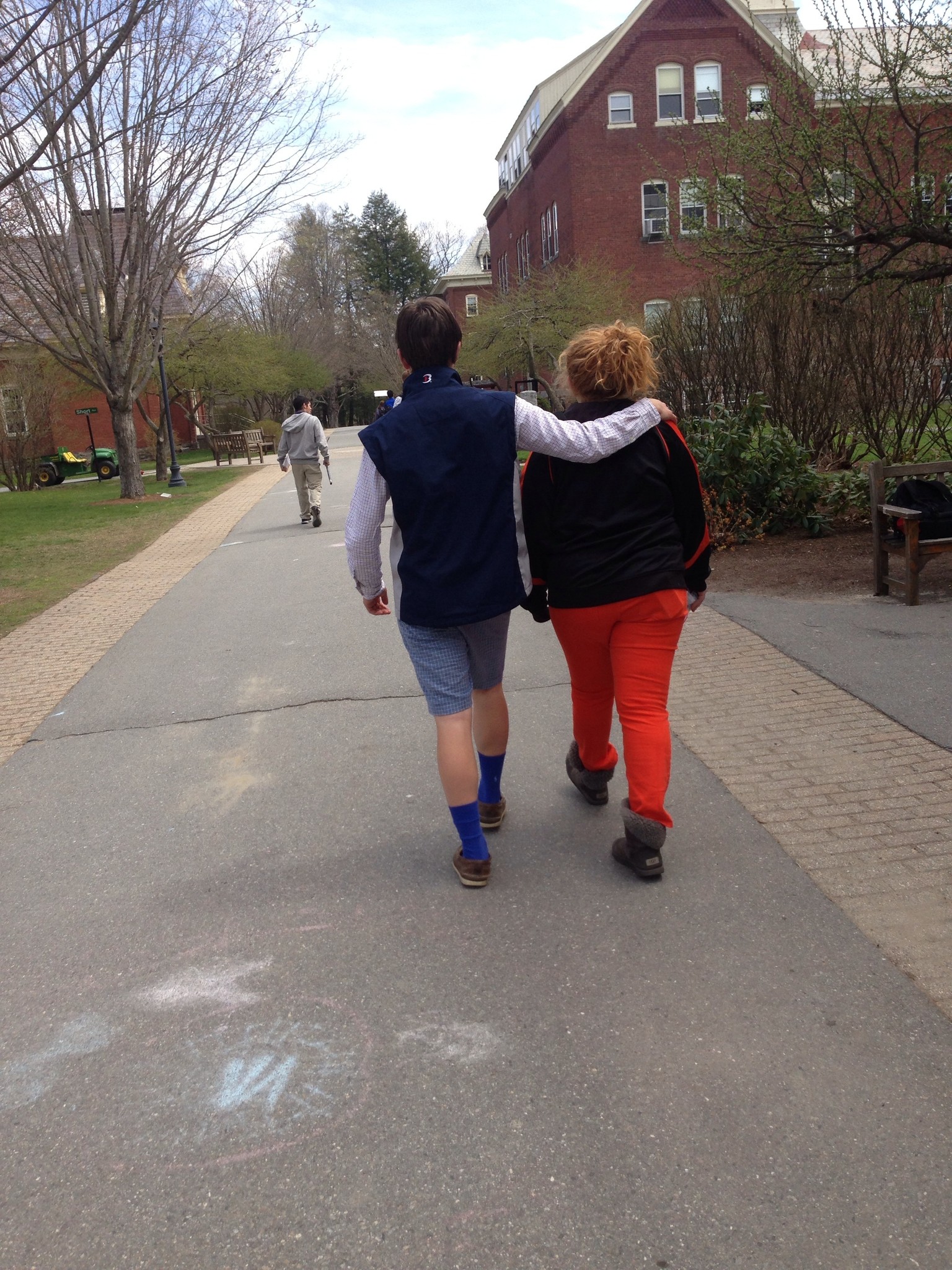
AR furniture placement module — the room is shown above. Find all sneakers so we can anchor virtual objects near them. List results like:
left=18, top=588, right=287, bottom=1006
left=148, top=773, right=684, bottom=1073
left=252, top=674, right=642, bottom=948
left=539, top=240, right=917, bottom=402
left=301, top=517, right=312, bottom=524
left=311, top=507, right=322, bottom=527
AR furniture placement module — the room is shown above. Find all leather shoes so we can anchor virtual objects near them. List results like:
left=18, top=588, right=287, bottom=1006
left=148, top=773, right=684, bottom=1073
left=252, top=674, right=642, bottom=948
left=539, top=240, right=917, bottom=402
left=452, top=844, right=492, bottom=886
left=477, top=791, right=506, bottom=829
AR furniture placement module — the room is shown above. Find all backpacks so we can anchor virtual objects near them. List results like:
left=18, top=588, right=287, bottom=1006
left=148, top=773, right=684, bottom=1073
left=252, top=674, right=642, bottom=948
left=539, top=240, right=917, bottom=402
left=886, top=479, right=933, bottom=542
left=384, top=405, right=393, bottom=414
left=917, top=480, right=952, bottom=538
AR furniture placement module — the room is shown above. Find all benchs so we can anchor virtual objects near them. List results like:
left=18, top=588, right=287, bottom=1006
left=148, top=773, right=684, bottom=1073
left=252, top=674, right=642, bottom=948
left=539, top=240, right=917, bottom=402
left=230, top=428, right=277, bottom=458
left=868, top=459, right=952, bottom=605
left=211, top=430, right=263, bottom=466
left=63, top=452, right=87, bottom=462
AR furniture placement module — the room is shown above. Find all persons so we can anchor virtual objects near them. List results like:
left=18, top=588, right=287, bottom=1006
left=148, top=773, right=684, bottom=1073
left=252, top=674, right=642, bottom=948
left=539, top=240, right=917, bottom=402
left=392, top=391, right=404, bottom=409
left=374, top=400, right=385, bottom=422
left=521, top=317, right=721, bottom=880
left=385, top=389, right=395, bottom=412
left=276, top=395, right=332, bottom=528
left=342, top=295, right=678, bottom=888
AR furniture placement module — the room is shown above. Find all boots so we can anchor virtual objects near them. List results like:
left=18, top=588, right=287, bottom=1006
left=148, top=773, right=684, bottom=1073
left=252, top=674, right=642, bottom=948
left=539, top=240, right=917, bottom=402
left=565, top=739, right=616, bottom=806
left=612, top=796, right=666, bottom=876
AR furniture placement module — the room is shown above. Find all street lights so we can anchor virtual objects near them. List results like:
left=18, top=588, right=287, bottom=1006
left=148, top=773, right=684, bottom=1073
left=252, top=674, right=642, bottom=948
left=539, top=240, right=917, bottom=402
left=144, top=318, right=187, bottom=487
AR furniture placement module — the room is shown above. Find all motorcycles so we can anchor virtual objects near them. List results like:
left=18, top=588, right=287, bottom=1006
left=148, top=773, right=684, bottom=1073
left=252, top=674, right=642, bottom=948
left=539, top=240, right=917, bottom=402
left=26, top=446, right=119, bottom=487
